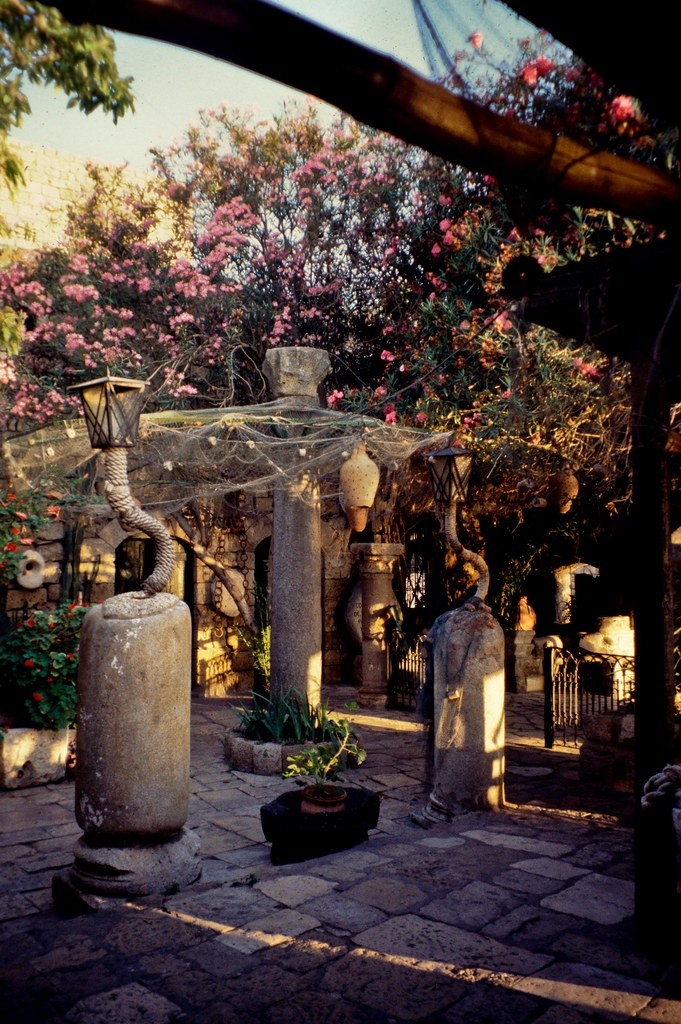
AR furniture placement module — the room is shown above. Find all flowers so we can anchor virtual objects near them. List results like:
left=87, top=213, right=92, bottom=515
left=15, top=600, right=103, bottom=728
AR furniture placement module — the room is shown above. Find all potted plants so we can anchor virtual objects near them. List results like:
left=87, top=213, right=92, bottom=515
left=282, top=701, right=366, bottom=814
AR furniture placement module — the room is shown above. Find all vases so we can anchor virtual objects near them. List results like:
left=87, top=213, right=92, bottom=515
left=0, top=726, right=69, bottom=788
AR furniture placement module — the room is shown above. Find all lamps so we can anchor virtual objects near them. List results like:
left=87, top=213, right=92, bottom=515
left=425, top=431, right=476, bottom=503
left=66, top=368, right=152, bottom=446
